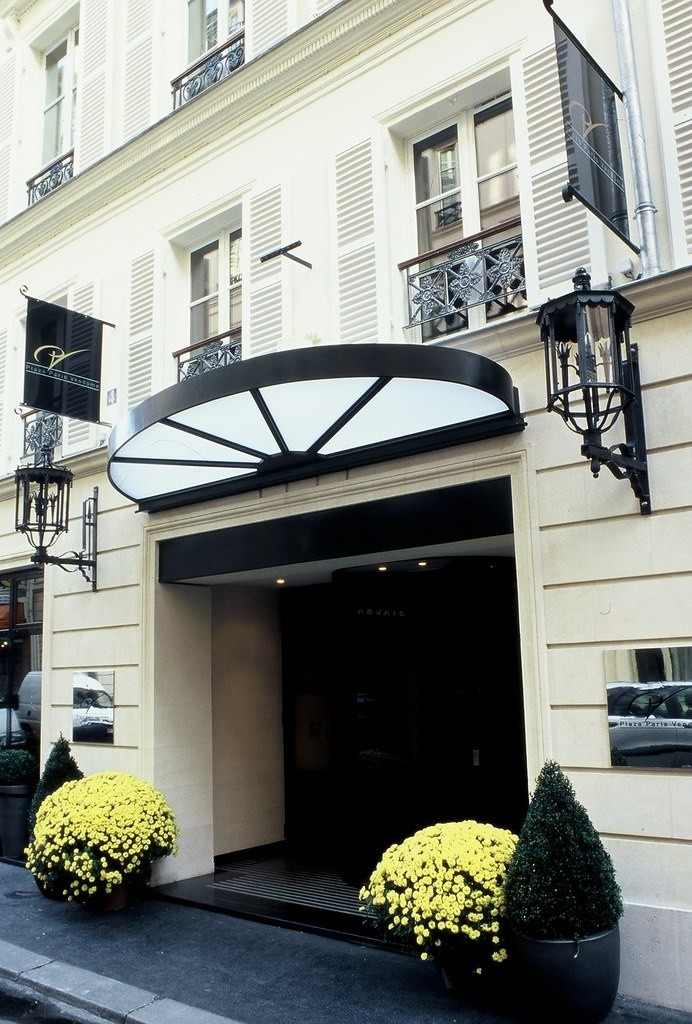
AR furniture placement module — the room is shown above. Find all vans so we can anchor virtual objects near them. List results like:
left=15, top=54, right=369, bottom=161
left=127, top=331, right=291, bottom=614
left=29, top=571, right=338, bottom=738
left=13, top=670, right=114, bottom=737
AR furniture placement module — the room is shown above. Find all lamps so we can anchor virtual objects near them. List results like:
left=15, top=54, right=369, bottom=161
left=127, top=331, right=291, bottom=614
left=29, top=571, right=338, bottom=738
left=537, top=266, right=653, bottom=516
left=13, top=441, right=98, bottom=593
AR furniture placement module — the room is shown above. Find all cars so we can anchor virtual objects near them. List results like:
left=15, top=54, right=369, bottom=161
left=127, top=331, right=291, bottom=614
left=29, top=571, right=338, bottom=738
left=0, top=695, right=33, bottom=753
left=605, top=679, right=692, bottom=770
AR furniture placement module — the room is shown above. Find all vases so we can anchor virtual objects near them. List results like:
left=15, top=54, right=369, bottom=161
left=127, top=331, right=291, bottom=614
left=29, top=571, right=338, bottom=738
left=441, top=953, right=472, bottom=989
left=94, top=881, right=128, bottom=910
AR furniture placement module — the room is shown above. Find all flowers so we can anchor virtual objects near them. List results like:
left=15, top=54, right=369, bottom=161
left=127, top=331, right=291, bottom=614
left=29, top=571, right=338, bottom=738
left=357, top=821, right=521, bottom=976
left=22, top=772, right=178, bottom=902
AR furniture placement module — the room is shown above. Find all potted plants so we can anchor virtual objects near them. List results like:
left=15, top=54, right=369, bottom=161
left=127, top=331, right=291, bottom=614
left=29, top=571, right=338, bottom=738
left=502, top=759, right=625, bottom=1024
left=0, top=749, right=38, bottom=858
left=27, top=730, right=84, bottom=899
